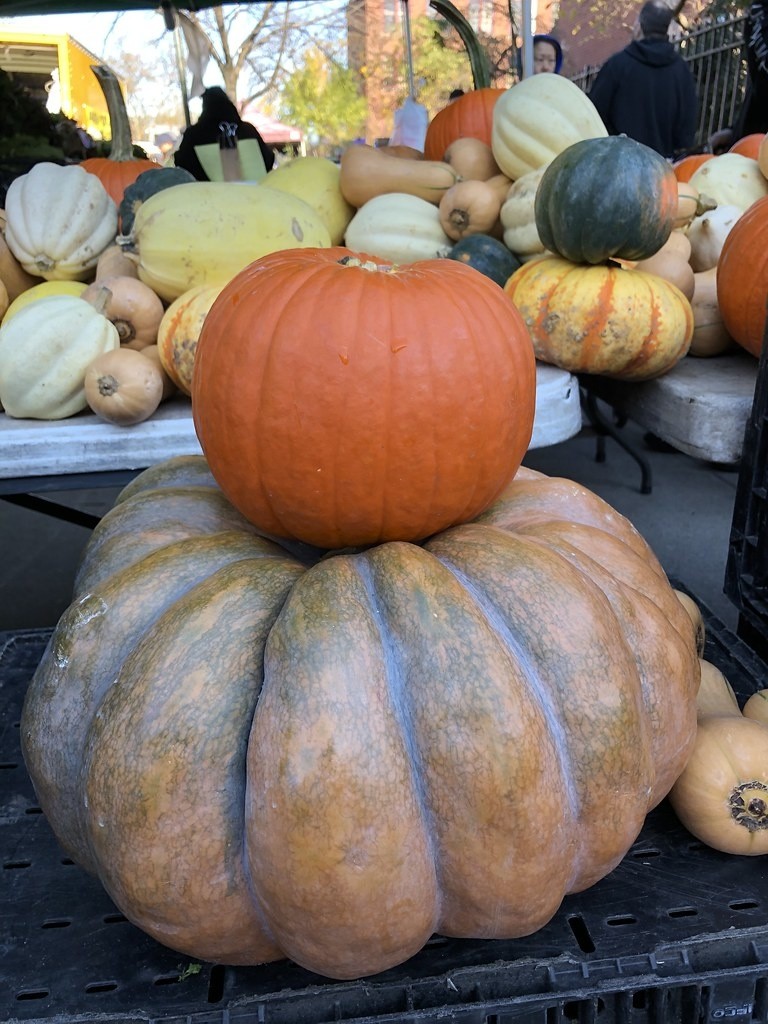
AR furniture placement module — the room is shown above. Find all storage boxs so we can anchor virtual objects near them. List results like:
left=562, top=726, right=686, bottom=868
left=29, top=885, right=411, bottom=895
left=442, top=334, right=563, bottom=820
left=0, top=574, right=768, bottom=1024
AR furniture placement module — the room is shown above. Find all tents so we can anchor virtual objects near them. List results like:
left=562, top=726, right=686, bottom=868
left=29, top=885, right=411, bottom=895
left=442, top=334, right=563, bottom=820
left=240, top=108, right=307, bottom=159
left=0, top=32, right=128, bottom=144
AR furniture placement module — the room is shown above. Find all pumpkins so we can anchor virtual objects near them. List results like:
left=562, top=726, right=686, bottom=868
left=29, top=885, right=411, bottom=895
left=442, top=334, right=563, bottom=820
left=0, top=74, right=767, bottom=982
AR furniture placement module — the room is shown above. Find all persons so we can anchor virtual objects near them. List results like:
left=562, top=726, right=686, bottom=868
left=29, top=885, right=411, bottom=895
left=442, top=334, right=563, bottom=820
left=589, top=0, right=700, bottom=163
left=174, top=85, right=275, bottom=183
left=705, top=0, right=768, bottom=158
left=517, top=34, right=564, bottom=80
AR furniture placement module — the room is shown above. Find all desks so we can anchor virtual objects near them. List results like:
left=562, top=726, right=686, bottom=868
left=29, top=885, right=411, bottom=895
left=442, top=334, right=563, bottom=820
left=0, top=360, right=585, bottom=530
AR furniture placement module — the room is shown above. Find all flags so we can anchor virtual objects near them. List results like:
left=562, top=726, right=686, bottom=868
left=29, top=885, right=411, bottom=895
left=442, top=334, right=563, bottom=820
left=178, top=13, right=211, bottom=101
